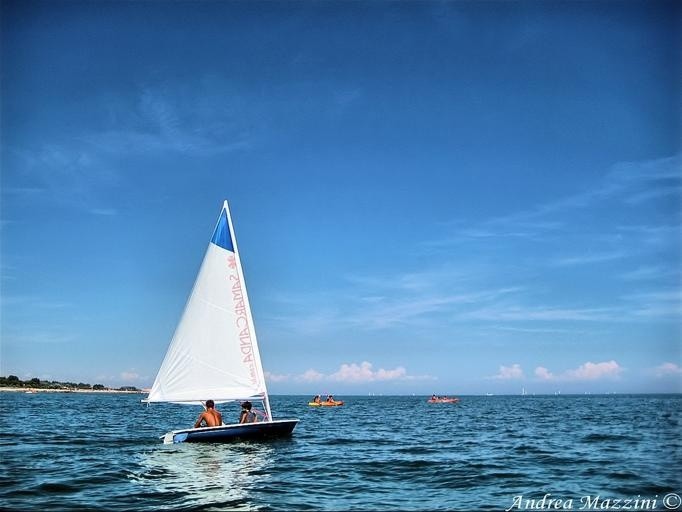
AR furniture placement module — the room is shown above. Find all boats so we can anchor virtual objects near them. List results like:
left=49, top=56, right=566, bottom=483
left=427, top=398, right=459, bottom=403
left=308, top=401, right=344, bottom=406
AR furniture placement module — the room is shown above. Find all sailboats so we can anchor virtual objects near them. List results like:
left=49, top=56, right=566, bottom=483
left=139, top=200, right=300, bottom=444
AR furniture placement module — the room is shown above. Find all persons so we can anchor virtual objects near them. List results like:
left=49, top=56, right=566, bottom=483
left=193, top=399, right=223, bottom=428
left=431, top=394, right=449, bottom=403
left=238, top=401, right=258, bottom=423
left=312, top=394, right=335, bottom=405
